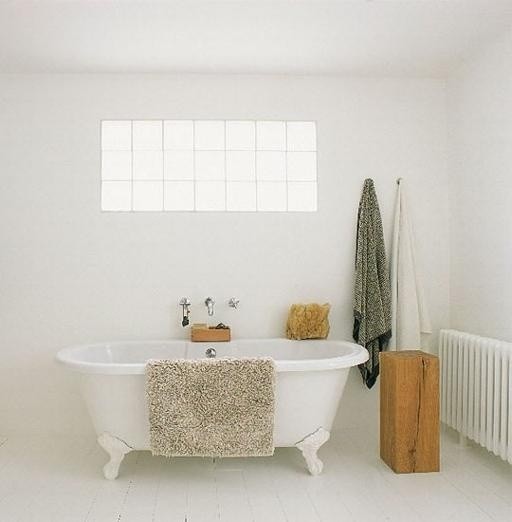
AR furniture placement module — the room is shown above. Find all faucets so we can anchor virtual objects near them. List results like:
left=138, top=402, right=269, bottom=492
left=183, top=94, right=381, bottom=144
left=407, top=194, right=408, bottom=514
left=203, top=298, right=216, bottom=316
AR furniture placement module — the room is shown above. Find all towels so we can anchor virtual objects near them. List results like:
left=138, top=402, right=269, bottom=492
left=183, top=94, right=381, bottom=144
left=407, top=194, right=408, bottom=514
left=389, top=179, right=432, bottom=352
left=144, top=356, right=275, bottom=457
left=351, top=177, right=392, bottom=391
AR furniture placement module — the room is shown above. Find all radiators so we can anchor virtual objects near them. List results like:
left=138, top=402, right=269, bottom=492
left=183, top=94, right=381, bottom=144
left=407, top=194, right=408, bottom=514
left=438, top=328, right=512, bottom=466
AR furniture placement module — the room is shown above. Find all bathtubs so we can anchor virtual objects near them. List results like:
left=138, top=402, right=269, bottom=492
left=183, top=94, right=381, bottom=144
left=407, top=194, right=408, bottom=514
left=54, top=339, right=370, bottom=482
